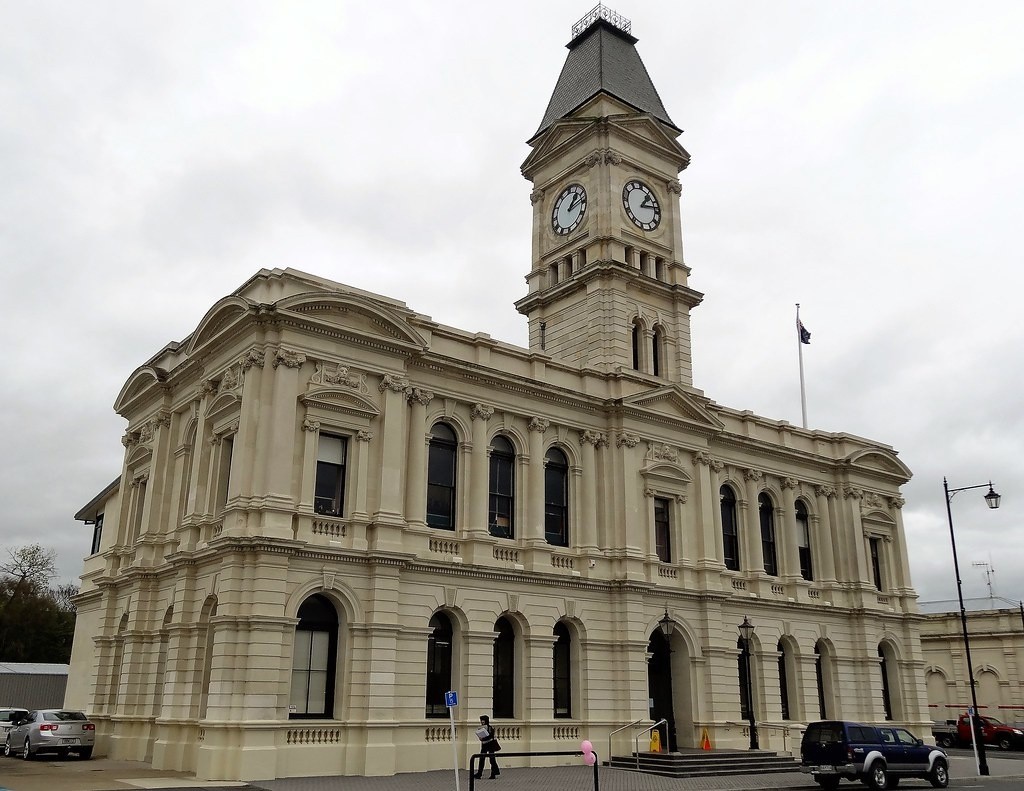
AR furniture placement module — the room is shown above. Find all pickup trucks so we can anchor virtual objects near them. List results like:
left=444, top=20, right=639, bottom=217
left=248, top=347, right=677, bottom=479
left=931, top=712, right=1024, bottom=750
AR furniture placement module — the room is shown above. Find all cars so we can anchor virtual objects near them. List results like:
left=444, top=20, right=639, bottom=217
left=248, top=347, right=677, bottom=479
left=0, top=707, right=29, bottom=749
left=4, top=710, right=96, bottom=761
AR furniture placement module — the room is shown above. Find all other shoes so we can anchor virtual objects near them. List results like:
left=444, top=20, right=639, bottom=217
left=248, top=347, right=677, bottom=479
left=489, top=775, right=495, bottom=778
left=474, top=774, right=480, bottom=779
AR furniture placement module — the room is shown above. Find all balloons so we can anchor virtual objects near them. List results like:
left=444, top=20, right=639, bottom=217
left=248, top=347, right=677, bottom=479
left=581, top=740, right=595, bottom=765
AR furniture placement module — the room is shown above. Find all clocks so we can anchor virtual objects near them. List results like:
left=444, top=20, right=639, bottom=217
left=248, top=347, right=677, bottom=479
left=548, top=183, right=587, bottom=236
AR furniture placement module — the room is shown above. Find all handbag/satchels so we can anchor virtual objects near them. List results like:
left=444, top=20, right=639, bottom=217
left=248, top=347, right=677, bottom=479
left=493, top=739, right=501, bottom=752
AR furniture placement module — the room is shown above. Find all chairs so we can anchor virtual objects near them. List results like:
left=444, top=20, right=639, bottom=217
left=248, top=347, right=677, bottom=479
left=882, top=734, right=890, bottom=741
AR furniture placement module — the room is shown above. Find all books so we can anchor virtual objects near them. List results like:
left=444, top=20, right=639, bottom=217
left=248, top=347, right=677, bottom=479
left=476, top=725, right=491, bottom=743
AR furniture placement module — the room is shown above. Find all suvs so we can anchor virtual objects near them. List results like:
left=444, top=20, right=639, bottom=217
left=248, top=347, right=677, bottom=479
left=798, top=720, right=950, bottom=789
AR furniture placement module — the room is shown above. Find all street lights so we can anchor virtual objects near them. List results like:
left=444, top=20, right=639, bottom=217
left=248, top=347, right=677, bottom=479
left=940, top=476, right=1002, bottom=776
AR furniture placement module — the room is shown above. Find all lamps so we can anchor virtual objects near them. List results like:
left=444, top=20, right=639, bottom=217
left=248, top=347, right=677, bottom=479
left=622, top=180, right=661, bottom=231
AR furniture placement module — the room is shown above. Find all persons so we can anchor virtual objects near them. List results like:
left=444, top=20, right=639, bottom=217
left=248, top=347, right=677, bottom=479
left=222, top=368, right=236, bottom=390
left=474, top=715, right=497, bottom=779
left=336, top=364, right=350, bottom=381
left=139, top=424, right=151, bottom=443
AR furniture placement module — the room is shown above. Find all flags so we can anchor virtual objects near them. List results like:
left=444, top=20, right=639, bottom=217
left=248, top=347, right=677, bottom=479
left=796, top=318, right=811, bottom=344
